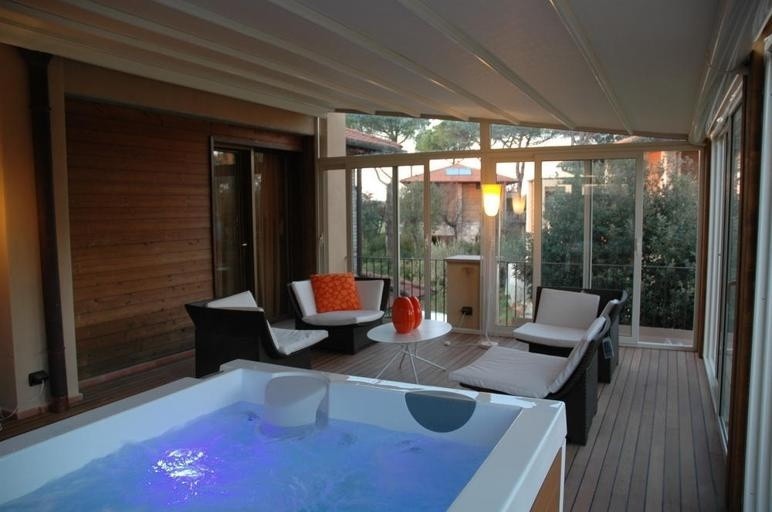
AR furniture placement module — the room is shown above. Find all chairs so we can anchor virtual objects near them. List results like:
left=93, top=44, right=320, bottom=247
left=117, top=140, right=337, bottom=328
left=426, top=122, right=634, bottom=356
left=450, top=285, right=630, bottom=447
left=184, top=277, right=391, bottom=378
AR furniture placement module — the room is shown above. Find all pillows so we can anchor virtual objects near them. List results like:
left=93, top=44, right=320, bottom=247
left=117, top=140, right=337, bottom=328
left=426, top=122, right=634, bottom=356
left=308, top=270, right=362, bottom=313
